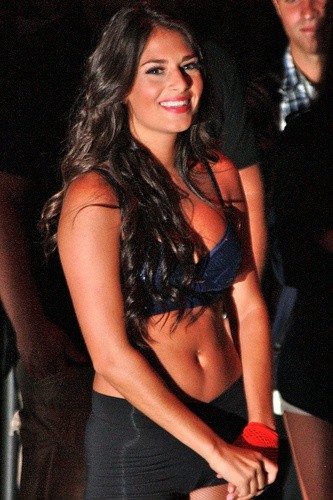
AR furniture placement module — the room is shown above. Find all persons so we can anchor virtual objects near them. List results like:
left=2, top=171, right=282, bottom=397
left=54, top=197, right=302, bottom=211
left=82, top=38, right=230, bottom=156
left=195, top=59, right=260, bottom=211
left=216, top=1, right=333, bottom=160
left=0, top=1, right=122, bottom=500
left=260, top=1, right=332, bottom=500
left=33, top=0, right=283, bottom=500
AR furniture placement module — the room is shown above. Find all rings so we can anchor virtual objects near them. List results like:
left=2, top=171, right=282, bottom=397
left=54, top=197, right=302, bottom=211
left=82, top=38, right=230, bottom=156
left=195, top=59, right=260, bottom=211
left=258, top=486, right=267, bottom=490
